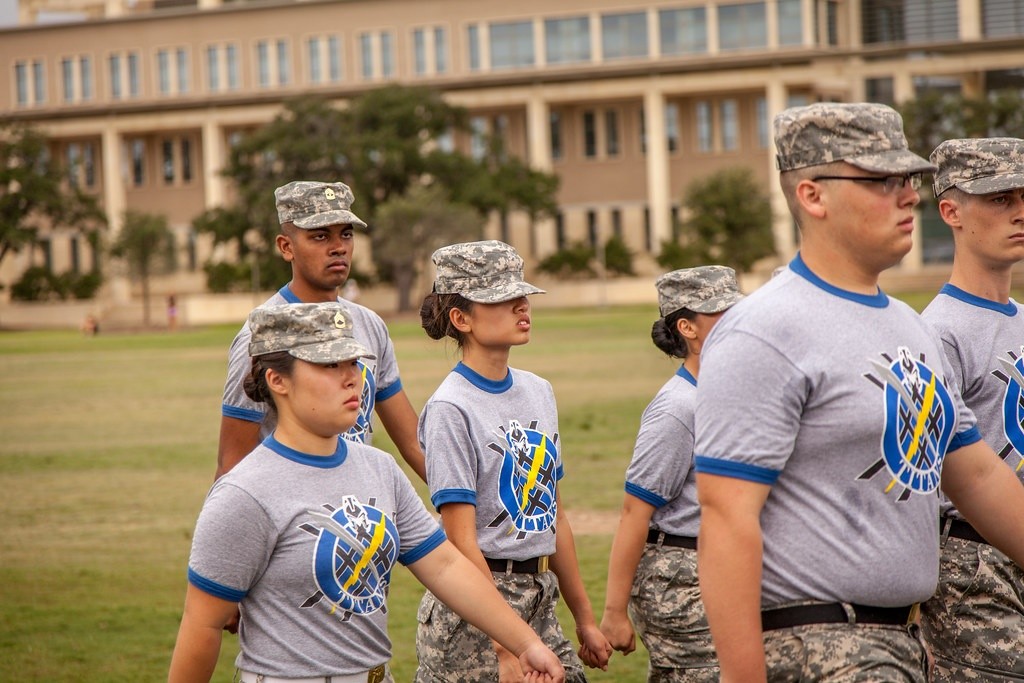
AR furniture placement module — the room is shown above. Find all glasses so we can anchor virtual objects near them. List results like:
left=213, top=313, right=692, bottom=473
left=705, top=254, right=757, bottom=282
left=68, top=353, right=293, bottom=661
left=812, top=171, right=924, bottom=194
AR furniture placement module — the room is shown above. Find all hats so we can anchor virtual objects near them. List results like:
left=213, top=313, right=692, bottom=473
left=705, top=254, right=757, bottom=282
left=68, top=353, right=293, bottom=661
left=654, top=264, right=747, bottom=317
left=431, top=239, right=548, bottom=304
left=772, top=102, right=939, bottom=173
left=246, top=301, right=378, bottom=365
left=929, top=137, right=1024, bottom=198
left=274, top=180, right=368, bottom=229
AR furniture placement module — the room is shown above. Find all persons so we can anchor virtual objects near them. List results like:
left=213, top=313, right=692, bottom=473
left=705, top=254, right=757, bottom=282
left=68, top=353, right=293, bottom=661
left=600, top=265, right=749, bottom=683
left=415, top=239, right=613, bottom=683
left=169, top=300, right=564, bottom=683
left=693, top=101, right=1024, bottom=683
left=214, top=180, right=426, bottom=634
left=920, top=137, right=1024, bottom=683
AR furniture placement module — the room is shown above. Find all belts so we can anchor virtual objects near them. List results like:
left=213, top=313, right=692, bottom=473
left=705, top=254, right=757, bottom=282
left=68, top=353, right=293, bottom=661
left=240, top=664, right=385, bottom=683
left=940, top=516, right=991, bottom=544
left=645, top=529, right=697, bottom=549
left=761, top=600, right=924, bottom=632
left=484, top=555, right=549, bottom=574
